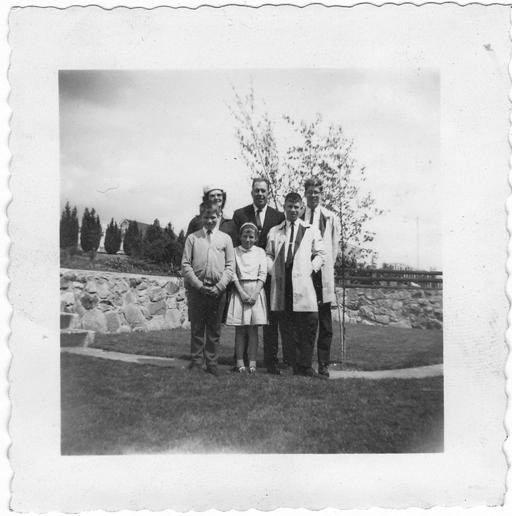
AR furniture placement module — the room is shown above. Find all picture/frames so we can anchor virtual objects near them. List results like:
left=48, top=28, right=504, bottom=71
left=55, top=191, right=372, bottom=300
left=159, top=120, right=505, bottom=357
left=3, top=1, right=512, bottom=515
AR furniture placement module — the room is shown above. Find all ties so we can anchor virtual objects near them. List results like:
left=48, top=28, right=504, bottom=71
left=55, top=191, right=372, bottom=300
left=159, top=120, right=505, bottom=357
left=286, top=222, right=295, bottom=267
left=256, top=209, right=263, bottom=230
left=310, top=209, right=314, bottom=224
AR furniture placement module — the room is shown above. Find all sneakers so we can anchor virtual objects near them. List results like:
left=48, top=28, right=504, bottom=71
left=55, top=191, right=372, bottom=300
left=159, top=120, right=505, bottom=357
left=295, top=369, right=313, bottom=377
left=189, top=364, right=202, bottom=373
left=283, top=361, right=293, bottom=369
left=235, top=367, right=246, bottom=373
left=250, top=368, right=256, bottom=374
left=207, top=366, right=217, bottom=375
left=319, top=364, right=329, bottom=377
left=267, top=368, right=281, bottom=375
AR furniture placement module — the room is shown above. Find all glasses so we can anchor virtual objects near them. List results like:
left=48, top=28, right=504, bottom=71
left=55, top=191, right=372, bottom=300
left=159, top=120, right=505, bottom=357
left=306, top=190, right=321, bottom=194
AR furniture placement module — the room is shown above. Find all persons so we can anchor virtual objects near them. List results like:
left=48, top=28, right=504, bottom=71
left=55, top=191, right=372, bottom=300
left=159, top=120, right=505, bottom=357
left=233, top=177, right=285, bottom=377
left=224, top=222, right=271, bottom=374
left=266, top=192, right=331, bottom=381
left=180, top=201, right=236, bottom=377
left=299, top=177, right=339, bottom=376
left=187, top=184, right=236, bottom=324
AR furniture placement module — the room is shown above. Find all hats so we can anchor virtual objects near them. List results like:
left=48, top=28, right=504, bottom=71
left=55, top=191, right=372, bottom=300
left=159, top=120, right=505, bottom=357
left=203, top=184, right=223, bottom=194
